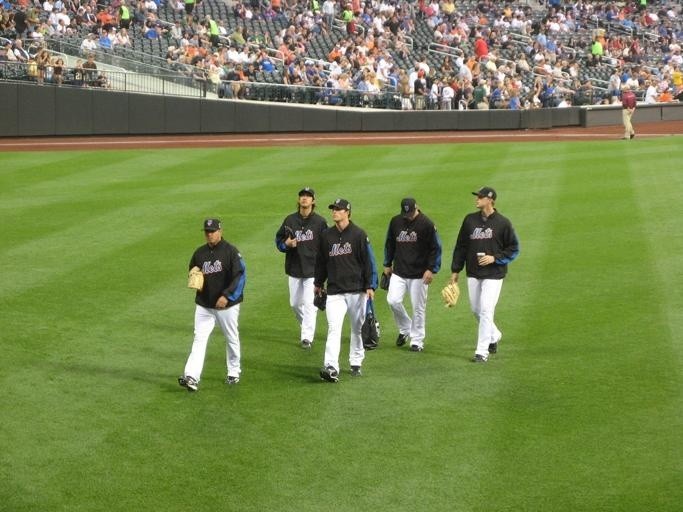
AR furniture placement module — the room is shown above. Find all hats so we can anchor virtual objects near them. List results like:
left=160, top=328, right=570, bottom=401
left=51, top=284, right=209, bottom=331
left=297, top=187, right=316, bottom=200
left=399, top=198, right=418, bottom=219
left=328, top=197, right=351, bottom=211
left=86, top=26, right=118, bottom=39
left=201, top=218, right=222, bottom=231
left=471, top=185, right=498, bottom=201
left=479, top=78, right=487, bottom=86
left=621, top=84, right=631, bottom=91
left=417, top=68, right=425, bottom=78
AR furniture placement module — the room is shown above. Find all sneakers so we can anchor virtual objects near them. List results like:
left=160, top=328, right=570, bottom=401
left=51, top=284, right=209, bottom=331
left=318, top=365, right=340, bottom=384
left=224, top=375, right=240, bottom=385
left=300, top=337, right=312, bottom=350
left=178, top=376, right=199, bottom=393
left=471, top=354, right=488, bottom=364
left=620, top=133, right=636, bottom=140
left=488, top=342, right=499, bottom=354
left=395, top=332, right=409, bottom=347
left=410, top=343, right=424, bottom=353
left=350, top=366, right=362, bottom=378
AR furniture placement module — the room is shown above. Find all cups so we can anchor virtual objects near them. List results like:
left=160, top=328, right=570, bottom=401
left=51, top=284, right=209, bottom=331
left=476, top=253, right=486, bottom=265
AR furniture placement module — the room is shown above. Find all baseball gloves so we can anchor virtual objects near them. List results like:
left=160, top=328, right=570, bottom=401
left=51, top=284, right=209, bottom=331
left=380, top=272, right=392, bottom=290
left=282, top=225, right=296, bottom=252
left=187, top=266, right=204, bottom=291
left=439, top=281, right=460, bottom=308
left=313, top=288, right=326, bottom=311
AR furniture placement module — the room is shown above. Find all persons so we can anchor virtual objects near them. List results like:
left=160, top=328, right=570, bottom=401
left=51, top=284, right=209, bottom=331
left=312, top=197, right=378, bottom=382
left=619, top=86, right=636, bottom=139
left=447, top=187, right=518, bottom=364
left=97, top=0, right=683, bottom=109
left=1, top=0, right=106, bottom=88
left=272, top=185, right=327, bottom=351
left=175, top=215, right=246, bottom=391
left=379, top=196, right=442, bottom=353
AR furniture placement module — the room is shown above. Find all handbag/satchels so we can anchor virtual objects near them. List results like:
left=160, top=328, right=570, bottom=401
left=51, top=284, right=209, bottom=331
left=361, top=294, right=381, bottom=352
